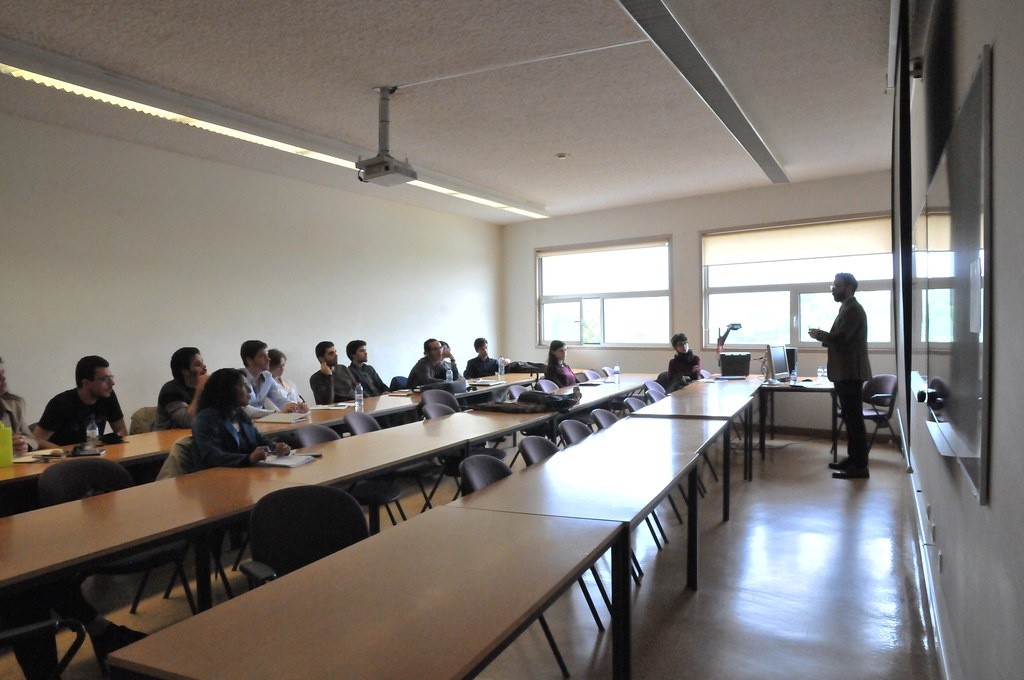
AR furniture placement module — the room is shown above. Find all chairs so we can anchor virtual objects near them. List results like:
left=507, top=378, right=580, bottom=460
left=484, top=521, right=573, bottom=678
left=156, top=434, right=260, bottom=599
left=643, top=380, right=668, bottom=396
left=657, top=371, right=670, bottom=390
left=421, top=403, right=484, bottom=514
left=423, top=389, right=488, bottom=492
left=602, top=367, right=636, bottom=398
left=345, top=411, right=434, bottom=521
left=583, top=370, right=628, bottom=418
left=42, top=457, right=200, bottom=616
left=239, top=486, right=368, bottom=589
left=645, top=389, right=719, bottom=493
left=624, top=398, right=707, bottom=508
left=591, top=409, right=684, bottom=543
left=700, top=370, right=745, bottom=440
left=555, top=419, right=662, bottom=576
left=456, top=454, right=605, bottom=680
left=830, top=375, right=903, bottom=456
left=521, top=436, right=641, bottom=616
left=508, top=385, right=565, bottom=468
left=536, top=378, right=584, bottom=449
left=297, top=425, right=396, bottom=528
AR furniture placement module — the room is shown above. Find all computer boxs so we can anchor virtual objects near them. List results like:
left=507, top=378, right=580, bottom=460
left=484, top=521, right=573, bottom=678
left=785, top=346, right=798, bottom=382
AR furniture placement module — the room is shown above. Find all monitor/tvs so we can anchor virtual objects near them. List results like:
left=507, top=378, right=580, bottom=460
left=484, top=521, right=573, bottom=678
left=763, top=345, right=790, bottom=384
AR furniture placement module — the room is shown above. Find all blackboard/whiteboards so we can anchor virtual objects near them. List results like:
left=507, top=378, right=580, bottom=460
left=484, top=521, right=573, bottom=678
left=911, top=40, right=993, bottom=506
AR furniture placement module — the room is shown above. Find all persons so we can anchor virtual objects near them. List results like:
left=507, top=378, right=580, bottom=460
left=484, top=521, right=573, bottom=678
left=0, top=357, right=41, bottom=456
left=463, top=338, right=511, bottom=441
left=239, top=340, right=309, bottom=418
left=407, top=339, right=486, bottom=447
left=265, top=349, right=299, bottom=409
left=151, top=346, right=210, bottom=472
left=34, top=355, right=128, bottom=449
left=668, top=333, right=701, bottom=384
left=312, top=341, right=397, bottom=481
left=192, top=367, right=290, bottom=468
left=345, top=341, right=391, bottom=397
left=0, top=496, right=147, bottom=680
left=808, top=273, right=873, bottom=478
left=545, top=340, right=580, bottom=388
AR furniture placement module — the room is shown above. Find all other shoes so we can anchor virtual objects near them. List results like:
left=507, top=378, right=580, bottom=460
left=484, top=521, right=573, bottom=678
left=832, top=464, right=869, bottom=480
left=828, top=458, right=849, bottom=470
left=98, top=625, right=150, bottom=649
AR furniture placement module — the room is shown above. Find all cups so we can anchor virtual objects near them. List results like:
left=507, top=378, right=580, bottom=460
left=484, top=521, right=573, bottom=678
left=0, top=422, right=12, bottom=466
left=573, top=387, right=580, bottom=397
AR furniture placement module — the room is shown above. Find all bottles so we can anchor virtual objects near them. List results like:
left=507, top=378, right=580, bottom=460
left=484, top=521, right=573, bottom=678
left=354, top=382, right=364, bottom=406
left=498, top=355, right=505, bottom=374
left=446, top=369, right=453, bottom=383
left=817, top=366, right=828, bottom=380
left=790, top=369, right=797, bottom=385
left=86, top=419, right=99, bottom=450
left=614, top=365, right=620, bottom=383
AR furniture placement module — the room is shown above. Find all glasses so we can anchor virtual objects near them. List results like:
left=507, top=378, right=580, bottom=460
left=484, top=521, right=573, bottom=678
left=427, top=346, right=443, bottom=352
left=445, top=348, right=452, bottom=352
left=831, top=284, right=849, bottom=289
left=89, top=375, right=114, bottom=382
left=672, top=342, right=688, bottom=347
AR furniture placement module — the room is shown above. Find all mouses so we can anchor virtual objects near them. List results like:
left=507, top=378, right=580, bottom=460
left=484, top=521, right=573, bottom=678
left=802, top=378, right=813, bottom=382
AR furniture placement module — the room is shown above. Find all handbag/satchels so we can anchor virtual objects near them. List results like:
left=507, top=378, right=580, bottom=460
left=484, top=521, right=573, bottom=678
left=505, top=361, right=547, bottom=373
left=412, top=380, right=466, bottom=394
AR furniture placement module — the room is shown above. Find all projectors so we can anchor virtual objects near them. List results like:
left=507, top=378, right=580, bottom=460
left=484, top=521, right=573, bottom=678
left=364, top=163, right=418, bottom=187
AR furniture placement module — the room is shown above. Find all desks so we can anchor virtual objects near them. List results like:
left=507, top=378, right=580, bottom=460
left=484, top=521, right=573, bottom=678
left=1, top=369, right=838, bottom=680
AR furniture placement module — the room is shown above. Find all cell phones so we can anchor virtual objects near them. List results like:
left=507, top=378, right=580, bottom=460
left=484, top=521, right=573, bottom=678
left=296, top=451, right=322, bottom=457
left=32, top=453, right=65, bottom=459
left=64, top=449, right=106, bottom=457
